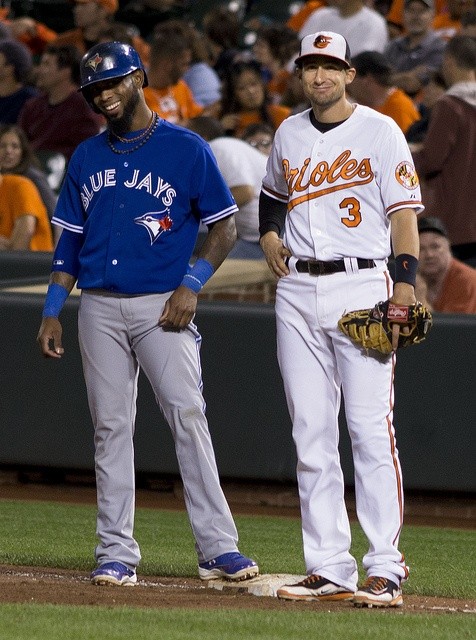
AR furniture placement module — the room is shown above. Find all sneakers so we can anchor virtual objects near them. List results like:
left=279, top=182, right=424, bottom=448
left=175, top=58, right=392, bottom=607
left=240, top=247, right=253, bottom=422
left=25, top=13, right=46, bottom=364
left=353, top=576, right=403, bottom=609
left=277, top=573, right=355, bottom=601
left=90, top=562, right=137, bottom=586
left=198, top=551, right=259, bottom=581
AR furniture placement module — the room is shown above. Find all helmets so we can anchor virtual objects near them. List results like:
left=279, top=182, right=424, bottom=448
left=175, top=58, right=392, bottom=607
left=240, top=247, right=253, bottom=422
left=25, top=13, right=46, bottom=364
left=77, top=41, right=148, bottom=114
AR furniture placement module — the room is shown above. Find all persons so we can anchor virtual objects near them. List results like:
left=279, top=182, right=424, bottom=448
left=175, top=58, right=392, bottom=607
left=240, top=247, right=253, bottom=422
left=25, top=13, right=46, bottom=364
left=64, top=2, right=129, bottom=42
left=20, top=44, right=100, bottom=169
left=207, top=54, right=291, bottom=137
left=137, top=28, right=207, bottom=135
left=386, top=3, right=448, bottom=53
left=36, top=38, right=259, bottom=587
left=301, top=0, right=391, bottom=59
left=0, top=124, right=57, bottom=229
left=403, top=211, right=475, bottom=318
left=2, top=165, right=52, bottom=253
left=258, top=30, right=430, bottom=607
left=413, top=26, right=476, bottom=250
left=342, top=44, right=417, bottom=141
left=200, top=133, right=283, bottom=248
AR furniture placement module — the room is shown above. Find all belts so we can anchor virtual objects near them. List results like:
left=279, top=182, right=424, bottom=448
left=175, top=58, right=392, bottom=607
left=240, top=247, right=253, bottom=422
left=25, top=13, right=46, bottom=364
left=283, top=255, right=377, bottom=276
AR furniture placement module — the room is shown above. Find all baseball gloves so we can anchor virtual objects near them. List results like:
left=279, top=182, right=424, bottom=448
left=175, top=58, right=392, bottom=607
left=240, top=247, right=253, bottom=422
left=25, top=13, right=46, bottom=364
left=337, top=301, right=434, bottom=353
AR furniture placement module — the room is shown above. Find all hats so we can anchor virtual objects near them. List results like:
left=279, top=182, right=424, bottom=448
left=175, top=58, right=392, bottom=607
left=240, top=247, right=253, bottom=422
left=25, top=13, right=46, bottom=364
left=295, top=31, right=352, bottom=68
left=416, top=216, right=448, bottom=237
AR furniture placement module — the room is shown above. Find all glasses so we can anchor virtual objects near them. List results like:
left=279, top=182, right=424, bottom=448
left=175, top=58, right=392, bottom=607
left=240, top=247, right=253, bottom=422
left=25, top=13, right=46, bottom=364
left=248, top=139, right=271, bottom=148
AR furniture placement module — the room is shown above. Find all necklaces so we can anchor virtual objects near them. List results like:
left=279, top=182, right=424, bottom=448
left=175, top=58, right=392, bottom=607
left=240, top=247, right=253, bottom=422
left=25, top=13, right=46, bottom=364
left=102, top=111, right=161, bottom=154
